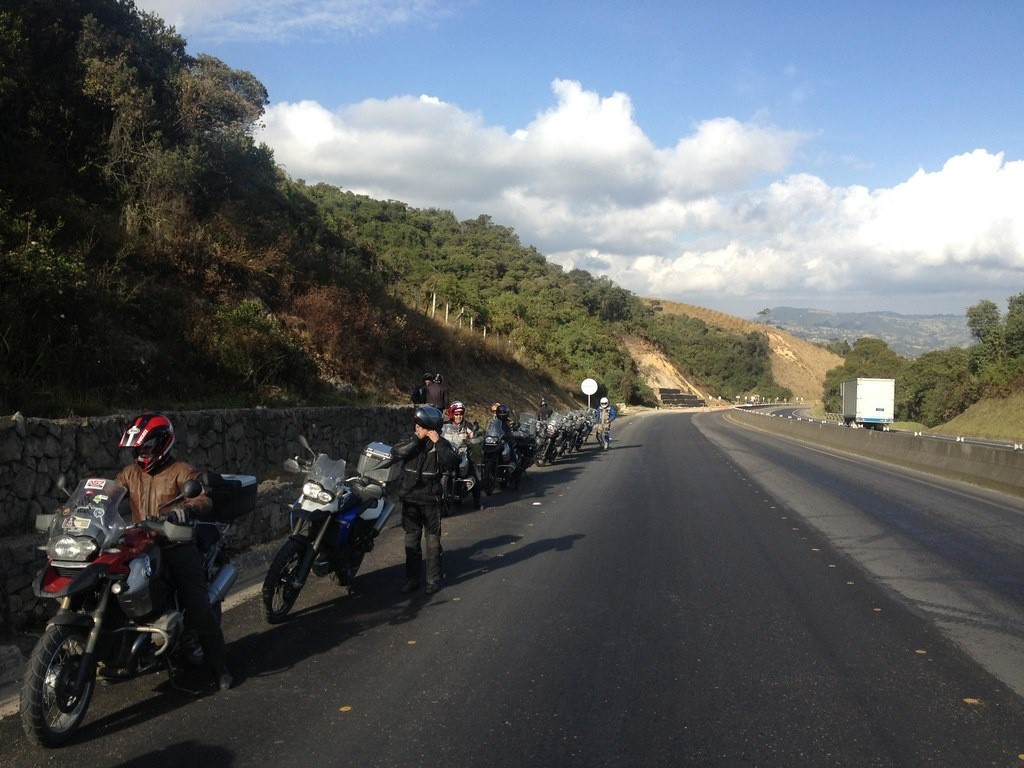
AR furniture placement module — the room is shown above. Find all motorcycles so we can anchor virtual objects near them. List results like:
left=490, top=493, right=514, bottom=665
left=16, top=472, right=261, bottom=742
left=434, top=421, right=476, bottom=519
left=261, top=437, right=411, bottom=618
left=517, top=409, right=597, bottom=466
left=474, top=418, right=535, bottom=496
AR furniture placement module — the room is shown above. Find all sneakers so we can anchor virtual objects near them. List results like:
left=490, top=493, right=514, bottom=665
left=214, top=649, right=233, bottom=691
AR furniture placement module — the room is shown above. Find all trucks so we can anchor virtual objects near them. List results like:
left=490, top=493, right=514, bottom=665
left=840, top=378, right=894, bottom=430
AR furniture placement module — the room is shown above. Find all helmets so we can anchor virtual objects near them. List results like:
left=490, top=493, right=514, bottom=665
left=433, top=373, right=442, bottom=384
left=496, top=404, right=509, bottom=420
left=600, top=396, right=608, bottom=408
left=414, top=406, right=443, bottom=433
left=117, top=414, right=175, bottom=474
left=448, top=401, right=465, bottom=420
left=423, top=373, right=433, bottom=384
left=540, top=400, right=548, bottom=407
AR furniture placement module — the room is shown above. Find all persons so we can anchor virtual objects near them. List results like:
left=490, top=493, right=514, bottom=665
left=594, top=396, right=617, bottom=451
left=52, top=410, right=233, bottom=691
left=440, top=400, right=487, bottom=511
left=535, top=398, right=554, bottom=428
left=420, top=373, right=450, bottom=413
left=483, top=400, right=500, bottom=432
left=410, top=372, right=434, bottom=405
left=478, top=404, right=524, bottom=493
left=390, top=406, right=463, bottom=594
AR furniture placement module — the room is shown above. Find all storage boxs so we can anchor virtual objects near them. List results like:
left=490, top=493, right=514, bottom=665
left=191, top=472, right=257, bottom=524
left=356, top=440, right=404, bottom=483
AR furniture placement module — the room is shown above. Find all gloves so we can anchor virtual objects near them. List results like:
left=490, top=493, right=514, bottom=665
left=160, top=512, right=177, bottom=526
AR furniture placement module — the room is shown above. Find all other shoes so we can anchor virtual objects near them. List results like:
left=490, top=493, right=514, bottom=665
left=474, top=501, right=484, bottom=511
left=600, top=443, right=609, bottom=450
left=402, top=583, right=416, bottom=593
left=425, top=584, right=440, bottom=594
